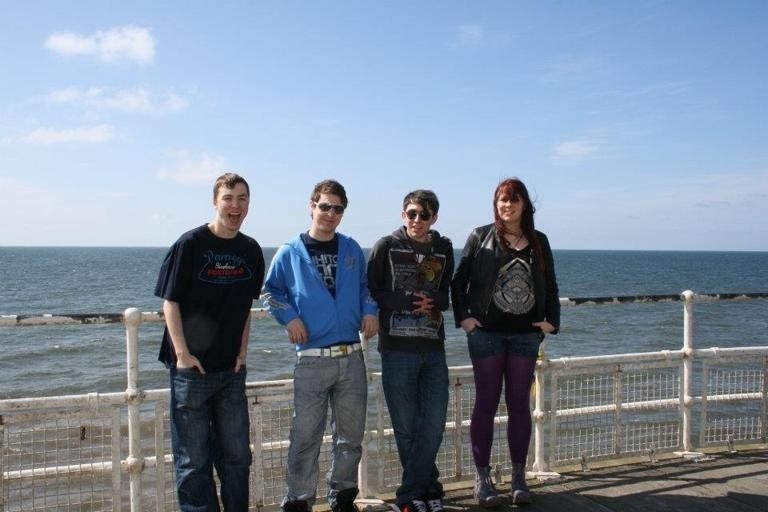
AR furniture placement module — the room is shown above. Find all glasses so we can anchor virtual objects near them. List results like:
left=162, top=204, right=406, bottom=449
left=405, top=208, right=435, bottom=221
left=313, top=201, right=345, bottom=215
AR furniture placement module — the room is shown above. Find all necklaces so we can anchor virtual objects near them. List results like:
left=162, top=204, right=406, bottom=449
left=499, top=233, right=525, bottom=250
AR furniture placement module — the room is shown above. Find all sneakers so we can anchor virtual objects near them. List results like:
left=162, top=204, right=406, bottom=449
left=393, top=495, right=446, bottom=512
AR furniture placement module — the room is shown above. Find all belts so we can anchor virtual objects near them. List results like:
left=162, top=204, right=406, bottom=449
left=297, top=342, right=362, bottom=359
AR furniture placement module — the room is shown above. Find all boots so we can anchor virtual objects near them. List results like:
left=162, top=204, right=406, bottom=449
left=473, top=465, right=501, bottom=508
left=508, top=463, right=534, bottom=504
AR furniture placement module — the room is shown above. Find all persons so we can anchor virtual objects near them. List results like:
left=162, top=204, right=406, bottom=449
left=448, top=173, right=561, bottom=509
left=154, top=170, right=266, bottom=512
left=365, top=189, right=458, bottom=511
left=257, top=178, right=381, bottom=511
